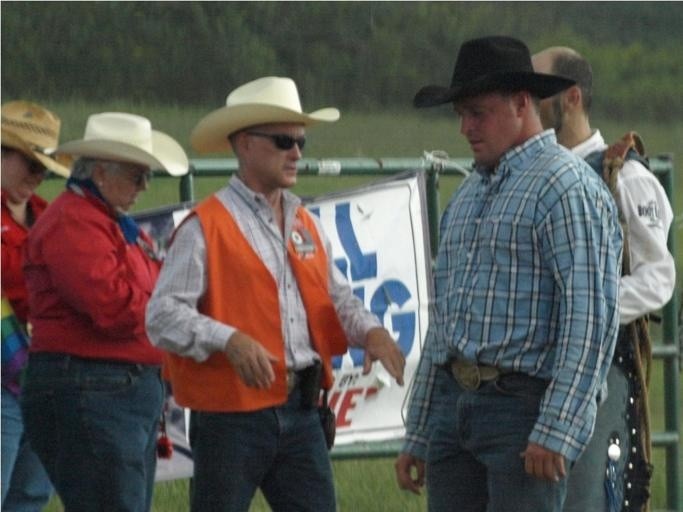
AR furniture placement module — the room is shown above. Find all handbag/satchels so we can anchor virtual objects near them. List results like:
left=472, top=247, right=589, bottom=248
left=317, top=404, right=337, bottom=450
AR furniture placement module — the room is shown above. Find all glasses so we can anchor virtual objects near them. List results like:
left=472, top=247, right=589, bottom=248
left=244, top=127, right=307, bottom=150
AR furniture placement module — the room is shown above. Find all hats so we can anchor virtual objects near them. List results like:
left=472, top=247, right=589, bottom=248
left=1, top=97, right=70, bottom=188
left=189, top=74, right=343, bottom=157
left=41, top=109, right=191, bottom=180
left=409, top=33, right=578, bottom=109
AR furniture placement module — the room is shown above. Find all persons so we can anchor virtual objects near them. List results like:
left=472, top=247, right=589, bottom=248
left=15, top=151, right=193, bottom=511
left=0, top=139, right=73, bottom=510
left=143, top=117, right=407, bottom=512
left=390, top=83, right=627, bottom=510
left=527, top=42, right=677, bottom=511
